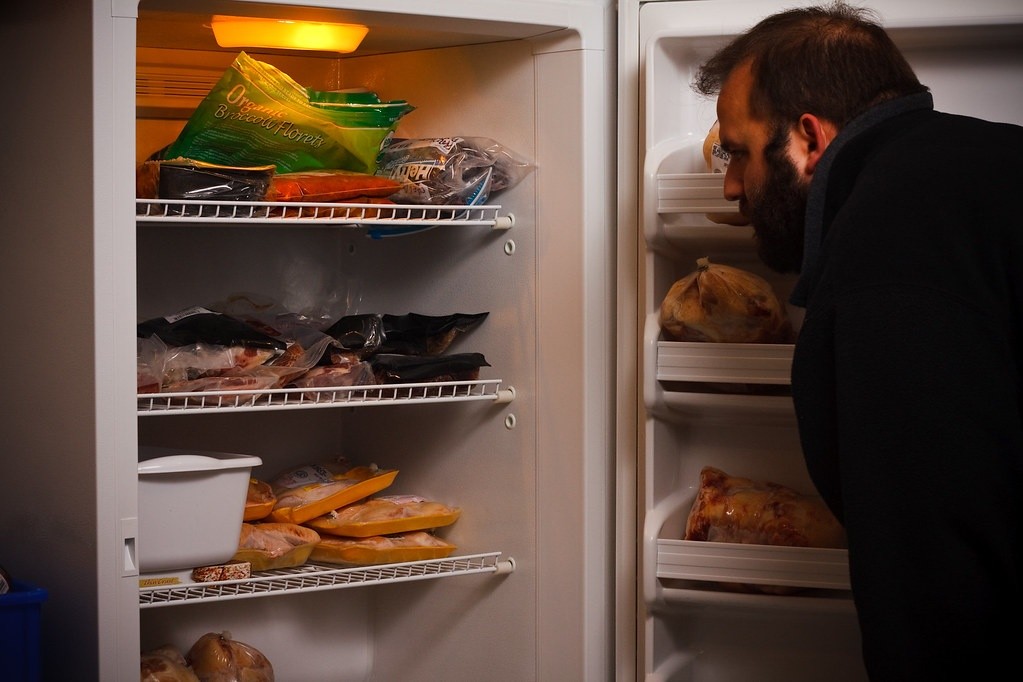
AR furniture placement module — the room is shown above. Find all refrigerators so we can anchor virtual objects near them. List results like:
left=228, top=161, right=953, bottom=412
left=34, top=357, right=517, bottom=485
left=0, top=1, right=1022, bottom=682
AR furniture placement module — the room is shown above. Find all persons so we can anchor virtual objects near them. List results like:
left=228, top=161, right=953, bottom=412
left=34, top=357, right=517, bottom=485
left=696, top=5, right=1023, bottom=682
